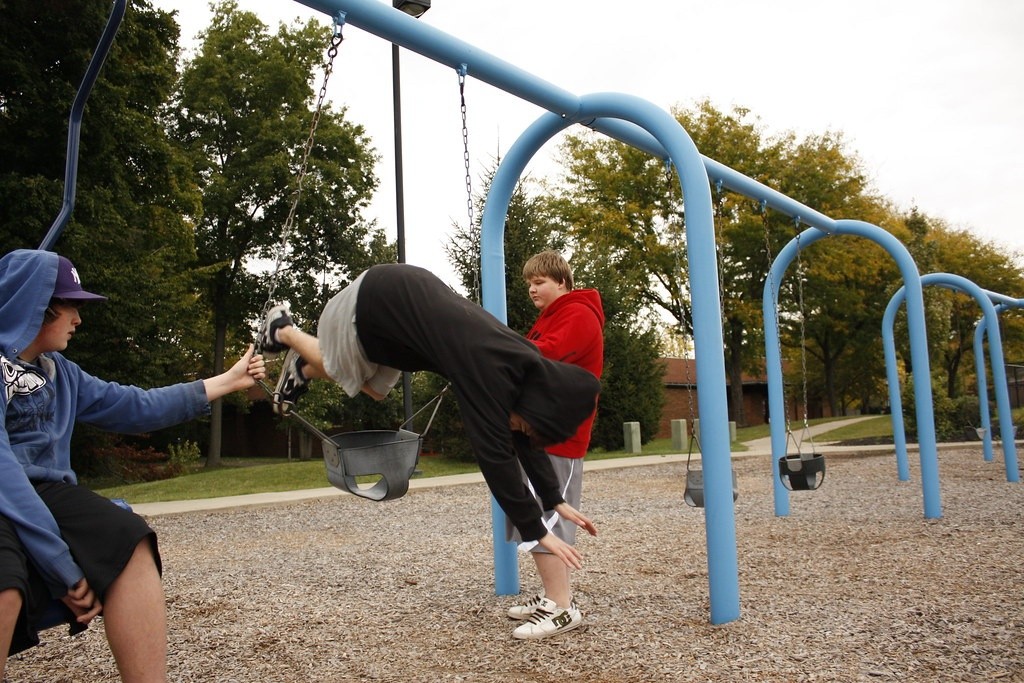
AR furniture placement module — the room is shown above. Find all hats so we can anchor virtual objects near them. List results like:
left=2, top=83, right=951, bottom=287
left=53, top=256, right=108, bottom=300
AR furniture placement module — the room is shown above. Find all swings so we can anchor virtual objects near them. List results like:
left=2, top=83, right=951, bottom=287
left=249, top=34, right=480, bottom=502
left=666, top=170, right=740, bottom=509
left=762, top=209, right=826, bottom=491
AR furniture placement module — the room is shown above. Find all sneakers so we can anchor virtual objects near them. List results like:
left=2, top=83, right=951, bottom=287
left=508, top=589, right=545, bottom=619
left=272, top=347, right=312, bottom=417
left=513, top=598, right=582, bottom=640
left=259, top=305, right=293, bottom=359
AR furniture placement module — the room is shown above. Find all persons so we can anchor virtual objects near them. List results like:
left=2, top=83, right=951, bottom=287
left=261, top=263, right=602, bottom=569
left=507, top=251, right=606, bottom=639
left=0, top=249, right=265, bottom=683
